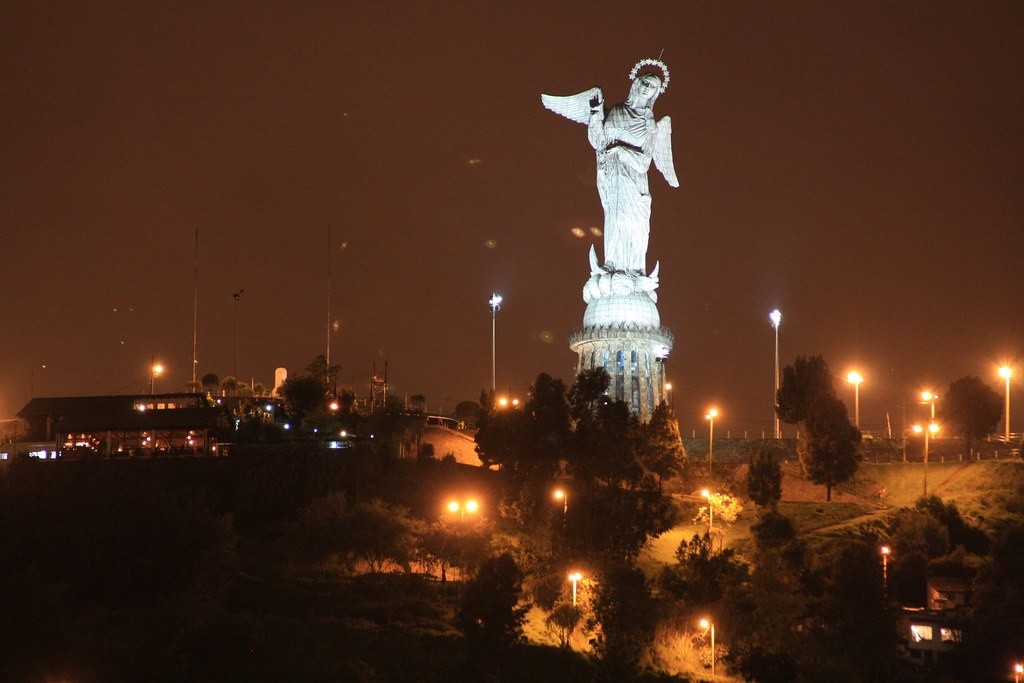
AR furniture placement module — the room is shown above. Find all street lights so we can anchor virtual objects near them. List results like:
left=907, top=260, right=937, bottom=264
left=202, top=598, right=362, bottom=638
left=707, top=407, right=718, bottom=478
left=448, top=497, right=478, bottom=522
left=880, top=545, right=889, bottom=590
left=149, top=356, right=163, bottom=395
left=489, top=291, right=504, bottom=392
left=921, top=423, right=941, bottom=496
left=847, top=369, right=862, bottom=428
left=702, top=487, right=714, bottom=532
left=698, top=619, right=714, bottom=675
left=998, top=366, right=1011, bottom=441
left=769, top=309, right=781, bottom=439
left=553, top=488, right=567, bottom=533
left=922, top=390, right=936, bottom=421
left=568, top=573, right=581, bottom=608
left=1014, top=663, right=1023, bottom=683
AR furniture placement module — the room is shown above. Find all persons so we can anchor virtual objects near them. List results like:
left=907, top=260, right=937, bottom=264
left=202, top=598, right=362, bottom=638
left=586, top=71, right=663, bottom=277
